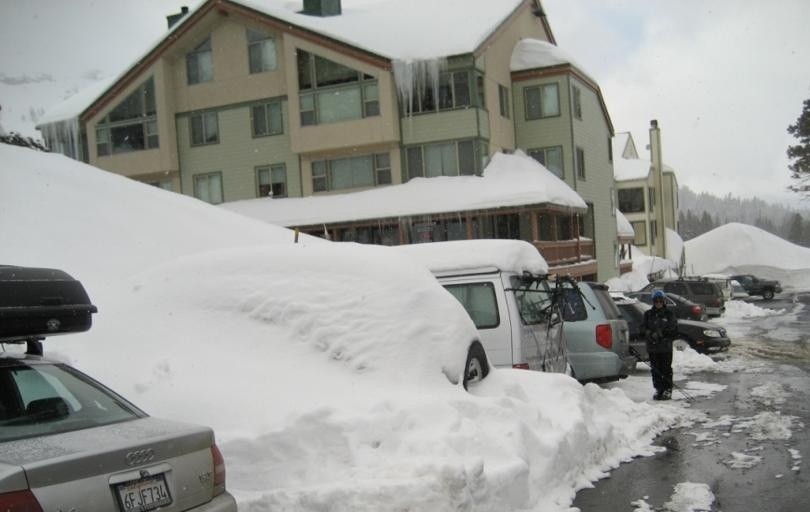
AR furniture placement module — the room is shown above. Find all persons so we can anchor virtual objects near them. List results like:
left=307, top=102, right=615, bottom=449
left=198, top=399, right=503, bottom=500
left=640, top=291, right=678, bottom=400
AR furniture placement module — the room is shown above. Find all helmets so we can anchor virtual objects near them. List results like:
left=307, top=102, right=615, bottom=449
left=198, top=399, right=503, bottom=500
left=652, top=290, right=666, bottom=300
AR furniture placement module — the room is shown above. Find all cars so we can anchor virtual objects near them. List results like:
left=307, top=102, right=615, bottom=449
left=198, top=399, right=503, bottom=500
left=0, top=261, right=237, bottom=510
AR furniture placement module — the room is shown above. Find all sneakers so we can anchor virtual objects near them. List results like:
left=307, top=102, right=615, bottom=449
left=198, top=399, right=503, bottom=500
left=653, top=387, right=672, bottom=400
